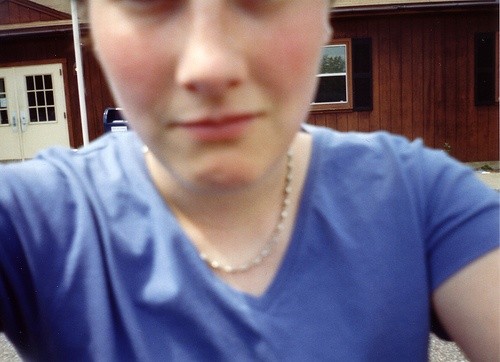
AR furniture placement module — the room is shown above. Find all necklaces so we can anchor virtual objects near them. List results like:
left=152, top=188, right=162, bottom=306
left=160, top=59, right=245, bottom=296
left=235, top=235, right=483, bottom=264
left=142, top=142, right=294, bottom=274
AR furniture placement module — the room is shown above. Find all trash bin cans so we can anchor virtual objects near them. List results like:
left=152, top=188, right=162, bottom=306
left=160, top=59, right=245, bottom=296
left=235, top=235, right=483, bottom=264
left=103, top=107, right=132, bottom=134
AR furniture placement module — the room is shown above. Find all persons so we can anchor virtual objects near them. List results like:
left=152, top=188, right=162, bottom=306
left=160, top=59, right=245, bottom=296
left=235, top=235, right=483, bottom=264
left=0, top=0, right=500, bottom=362
